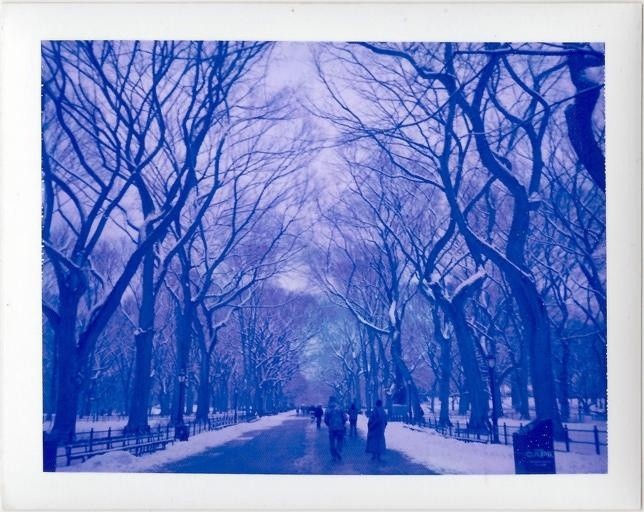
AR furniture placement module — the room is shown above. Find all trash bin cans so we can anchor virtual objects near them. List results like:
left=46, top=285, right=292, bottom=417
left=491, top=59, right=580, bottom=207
left=175, top=424, right=190, bottom=441
left=43, top=439, right=60, bottom=472
left=512, top=417, right=556, bottom=474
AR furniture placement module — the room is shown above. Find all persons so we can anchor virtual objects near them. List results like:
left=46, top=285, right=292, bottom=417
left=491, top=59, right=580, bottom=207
left=348, top=402, right=359, bottom=433
left=295, top=401, right=323, bottom=431
left=365, top=398, right=389, bottom=461
left=323, top=395, right=348, bottom=464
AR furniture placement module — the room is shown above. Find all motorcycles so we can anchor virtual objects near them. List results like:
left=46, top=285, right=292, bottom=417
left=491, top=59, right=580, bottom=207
left=309, top=411, right=315, bottom=423
